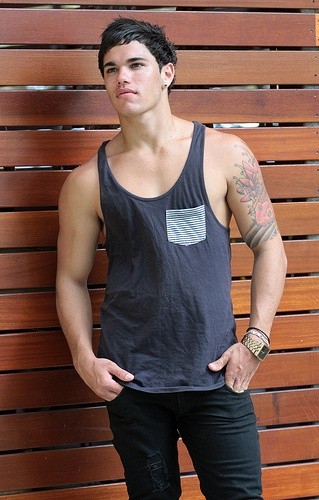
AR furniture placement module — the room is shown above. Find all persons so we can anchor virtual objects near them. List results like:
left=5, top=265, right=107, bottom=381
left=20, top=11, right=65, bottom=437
left=53, top=17, right=288, bottom=500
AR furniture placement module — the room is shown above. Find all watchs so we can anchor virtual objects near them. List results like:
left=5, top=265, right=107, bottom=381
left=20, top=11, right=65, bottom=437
left=241, top=335, right=270, bottom=361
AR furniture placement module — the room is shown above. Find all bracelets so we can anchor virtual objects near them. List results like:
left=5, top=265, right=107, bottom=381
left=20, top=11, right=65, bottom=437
left=243, top=329, right=269, bottom=347
left=246, top=327, right=271, bottom=345
